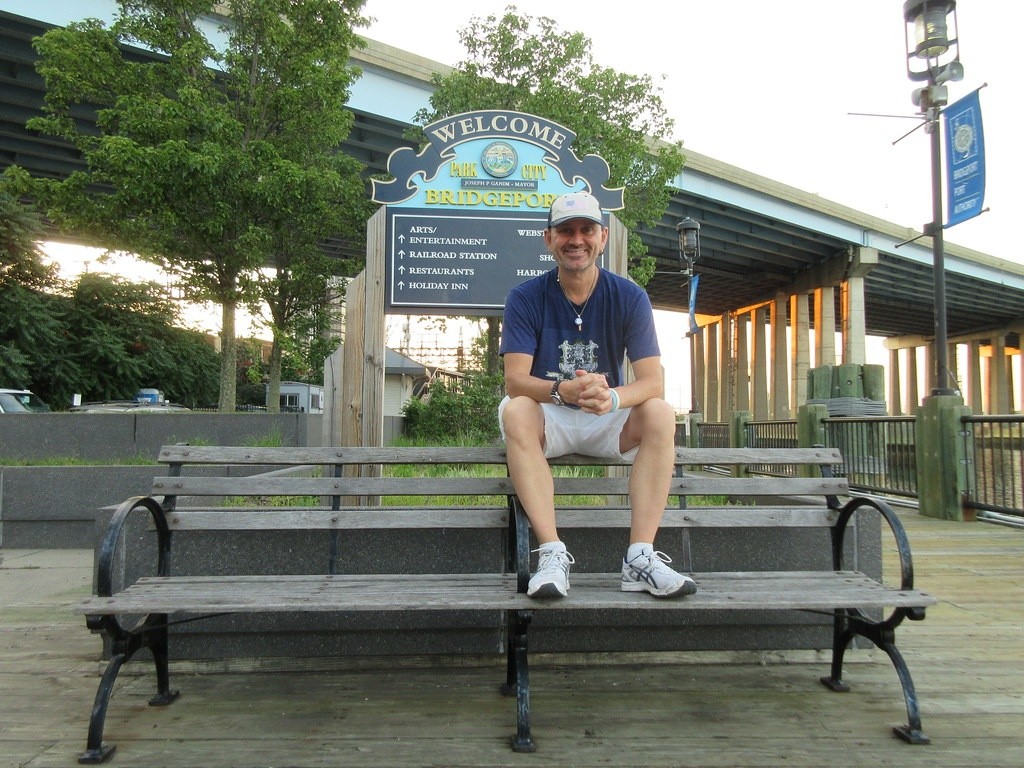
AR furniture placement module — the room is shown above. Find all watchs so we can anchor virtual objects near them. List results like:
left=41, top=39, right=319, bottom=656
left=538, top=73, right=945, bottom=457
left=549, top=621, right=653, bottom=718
left=551, top=380, right=567, bottom=406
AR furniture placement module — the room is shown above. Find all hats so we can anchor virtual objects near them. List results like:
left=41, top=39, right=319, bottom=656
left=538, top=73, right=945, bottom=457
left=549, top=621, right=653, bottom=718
left=547, top=192, right=605, bottom=227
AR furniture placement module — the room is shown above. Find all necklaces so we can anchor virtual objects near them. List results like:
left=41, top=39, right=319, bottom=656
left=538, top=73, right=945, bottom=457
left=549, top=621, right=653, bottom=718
left=557, top=268, right=598, bottom=331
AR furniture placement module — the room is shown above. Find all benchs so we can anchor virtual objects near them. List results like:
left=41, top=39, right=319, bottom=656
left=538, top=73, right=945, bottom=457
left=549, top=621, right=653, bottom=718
left=73, top=449, right=939, bottom=764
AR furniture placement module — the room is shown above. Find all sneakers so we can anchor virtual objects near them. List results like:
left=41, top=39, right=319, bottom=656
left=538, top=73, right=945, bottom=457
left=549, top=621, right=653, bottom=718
left=620, top=551, right=697, bottom=599
left=526, top=546, right=576, bottom=598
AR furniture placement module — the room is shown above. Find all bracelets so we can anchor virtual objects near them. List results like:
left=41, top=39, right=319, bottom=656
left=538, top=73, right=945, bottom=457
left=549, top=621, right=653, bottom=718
left=610, top=387, right=620, bottom=412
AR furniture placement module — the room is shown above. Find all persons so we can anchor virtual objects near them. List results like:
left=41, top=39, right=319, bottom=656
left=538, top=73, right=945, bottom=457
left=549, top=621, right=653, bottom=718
left=498, top=193, right=698, bottom=599
